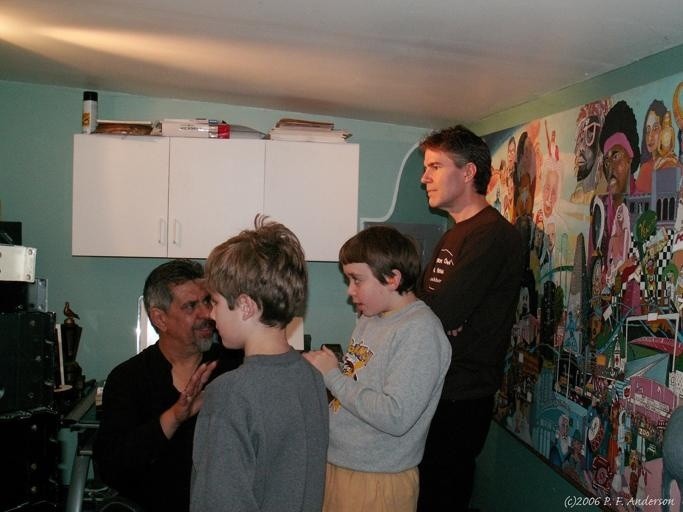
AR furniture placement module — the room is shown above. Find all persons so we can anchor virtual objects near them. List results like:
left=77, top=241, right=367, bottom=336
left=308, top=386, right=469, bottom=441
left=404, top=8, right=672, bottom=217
left=88, top=262, right=246, bottom=511
left=485, top=99, right=681, bottom=512
left=183, top=212, right=332, bottom=511
left=414, top=122, right=527, bottom=512
left=299, top=223, right=454, bottom=511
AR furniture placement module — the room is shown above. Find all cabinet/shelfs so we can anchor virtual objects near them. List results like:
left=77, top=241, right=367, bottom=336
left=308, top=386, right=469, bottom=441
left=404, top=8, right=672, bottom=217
left=73, top=133, right=360, bottom=264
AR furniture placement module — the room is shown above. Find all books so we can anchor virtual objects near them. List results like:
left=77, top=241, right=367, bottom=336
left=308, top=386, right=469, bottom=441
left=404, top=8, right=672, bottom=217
left=265, top=116, right=354, bottom=142
left=93, top=118, right=153, bottom=128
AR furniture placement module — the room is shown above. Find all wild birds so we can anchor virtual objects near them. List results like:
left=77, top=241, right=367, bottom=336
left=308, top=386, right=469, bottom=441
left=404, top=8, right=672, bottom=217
left=62, top=301, right=81, bottom=321
